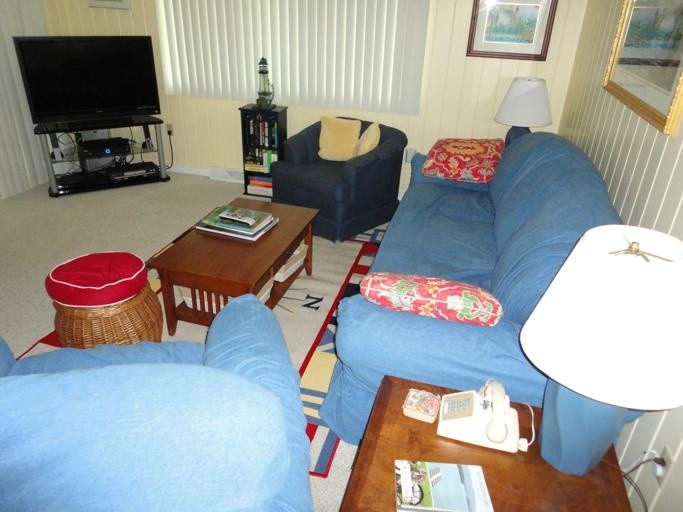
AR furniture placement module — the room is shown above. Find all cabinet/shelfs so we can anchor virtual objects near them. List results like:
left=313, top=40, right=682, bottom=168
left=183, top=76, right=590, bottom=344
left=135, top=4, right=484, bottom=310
left=238, top=103, right=289, bottom=199
left=31, top=113, right=174, bottom=199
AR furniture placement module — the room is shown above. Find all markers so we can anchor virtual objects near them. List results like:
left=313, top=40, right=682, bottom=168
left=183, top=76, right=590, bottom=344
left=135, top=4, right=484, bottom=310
left=123, top=170, right=150, bottom=176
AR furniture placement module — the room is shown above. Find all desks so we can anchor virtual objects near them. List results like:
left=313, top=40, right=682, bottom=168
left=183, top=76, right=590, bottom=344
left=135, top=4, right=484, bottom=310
left=335, top=368, right=636, bottom=512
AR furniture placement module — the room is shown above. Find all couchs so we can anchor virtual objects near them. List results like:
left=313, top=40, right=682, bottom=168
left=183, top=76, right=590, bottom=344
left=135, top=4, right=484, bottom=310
left=313, top=133, right=642, bottom=445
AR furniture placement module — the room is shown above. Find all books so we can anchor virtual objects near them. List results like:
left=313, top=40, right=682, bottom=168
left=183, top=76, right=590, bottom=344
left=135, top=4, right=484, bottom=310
left=244, top=115, right=279, bottom=196
left=394, top=458, right=496, bottom=512
left=195, top=205, right=279, bottom=242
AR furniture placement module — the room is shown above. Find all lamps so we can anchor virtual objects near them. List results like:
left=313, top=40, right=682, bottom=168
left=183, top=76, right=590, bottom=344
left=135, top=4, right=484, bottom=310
left=519, top=224, right=683, bottom=482
left=492, top=75, right=553, bottom=148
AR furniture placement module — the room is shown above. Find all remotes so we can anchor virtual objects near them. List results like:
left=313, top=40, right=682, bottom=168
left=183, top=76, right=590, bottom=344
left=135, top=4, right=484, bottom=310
left=219, top=211, right=255, bottom=227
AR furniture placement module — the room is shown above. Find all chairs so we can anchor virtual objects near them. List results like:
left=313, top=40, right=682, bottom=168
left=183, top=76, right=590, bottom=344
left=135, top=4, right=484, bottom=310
left=0, top=285, right=323, bottom=512
left=269, top=112, right=409, bottom=248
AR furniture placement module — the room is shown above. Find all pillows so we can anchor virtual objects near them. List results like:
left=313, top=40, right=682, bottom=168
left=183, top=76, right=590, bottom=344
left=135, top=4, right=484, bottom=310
left=356, top=121, right=383, bottom=157
left=358, top=269, right=505, bottom=329
left=421, top=135, right=506, bottom=187
left=315, top=115, right=362, bottom=162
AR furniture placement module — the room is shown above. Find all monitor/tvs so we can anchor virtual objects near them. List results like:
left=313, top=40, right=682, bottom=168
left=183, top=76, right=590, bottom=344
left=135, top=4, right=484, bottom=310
left=12, top=36, right=162, bottom=127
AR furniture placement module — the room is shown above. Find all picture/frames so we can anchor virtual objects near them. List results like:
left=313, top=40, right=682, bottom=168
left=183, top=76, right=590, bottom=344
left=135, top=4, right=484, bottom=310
left=464, top=0, right=560, bottom=64
left=597, top=0, right=683, bottom=141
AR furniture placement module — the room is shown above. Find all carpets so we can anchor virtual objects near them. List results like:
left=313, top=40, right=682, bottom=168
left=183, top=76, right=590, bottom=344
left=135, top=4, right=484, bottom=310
left=15, top=196, right=390, bottom=478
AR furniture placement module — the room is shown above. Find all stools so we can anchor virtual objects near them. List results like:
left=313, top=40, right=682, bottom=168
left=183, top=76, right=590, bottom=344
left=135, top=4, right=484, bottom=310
left=41, top=249, right=164, bottom=354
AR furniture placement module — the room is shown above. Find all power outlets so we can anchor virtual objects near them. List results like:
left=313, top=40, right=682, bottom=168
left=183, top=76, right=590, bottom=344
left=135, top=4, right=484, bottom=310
left=650, top=444, right=676, bottom=486
left=165, top=123, right=175, bottom=139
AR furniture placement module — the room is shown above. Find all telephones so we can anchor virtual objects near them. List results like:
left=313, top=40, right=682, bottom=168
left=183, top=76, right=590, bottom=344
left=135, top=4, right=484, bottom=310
left=436, top=380, right=520, bottom=454
